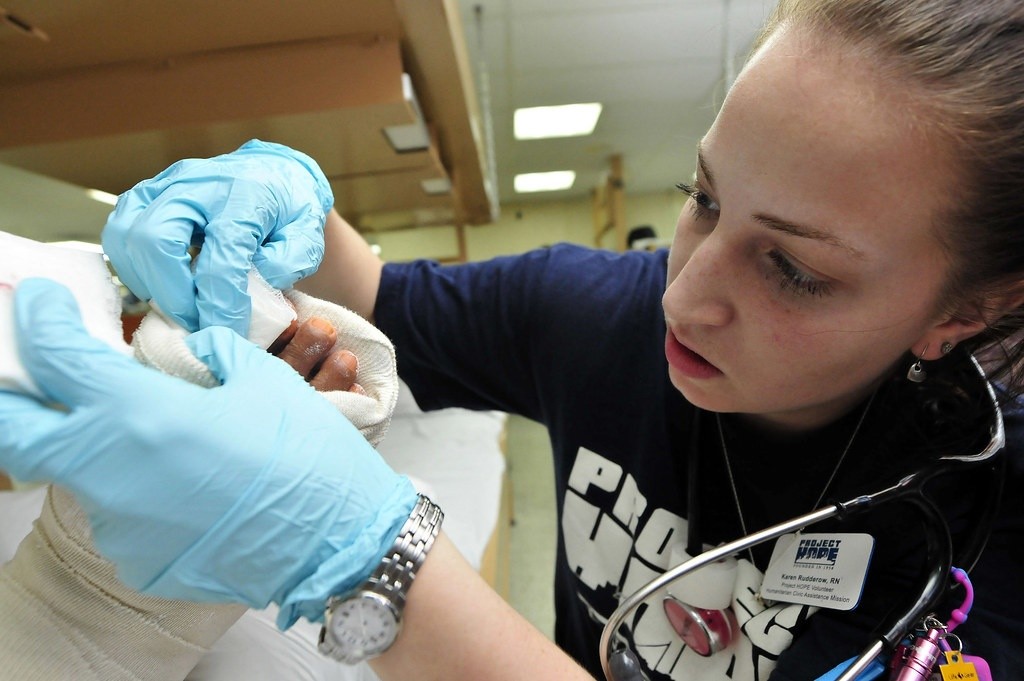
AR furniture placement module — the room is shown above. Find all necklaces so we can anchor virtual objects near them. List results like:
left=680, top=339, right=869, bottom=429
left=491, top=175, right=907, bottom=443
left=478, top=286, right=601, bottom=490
left=718, top=412, right=866, bottom=607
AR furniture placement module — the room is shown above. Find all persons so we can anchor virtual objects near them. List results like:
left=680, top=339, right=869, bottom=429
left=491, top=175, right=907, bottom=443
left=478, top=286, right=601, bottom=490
left=1, top=0, right=1023, bottom=677
left=2, top=233, right=368, bottom=681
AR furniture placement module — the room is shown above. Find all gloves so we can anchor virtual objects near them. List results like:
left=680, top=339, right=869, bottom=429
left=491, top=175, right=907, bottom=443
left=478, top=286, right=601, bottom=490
left=0, top=277, right=418, bottom=632
left=102, top=138, right=335, bottom=338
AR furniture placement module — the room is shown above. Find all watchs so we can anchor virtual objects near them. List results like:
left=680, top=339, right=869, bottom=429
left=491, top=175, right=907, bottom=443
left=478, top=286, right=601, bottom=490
left=316, top=492, right=445, bottom=664
